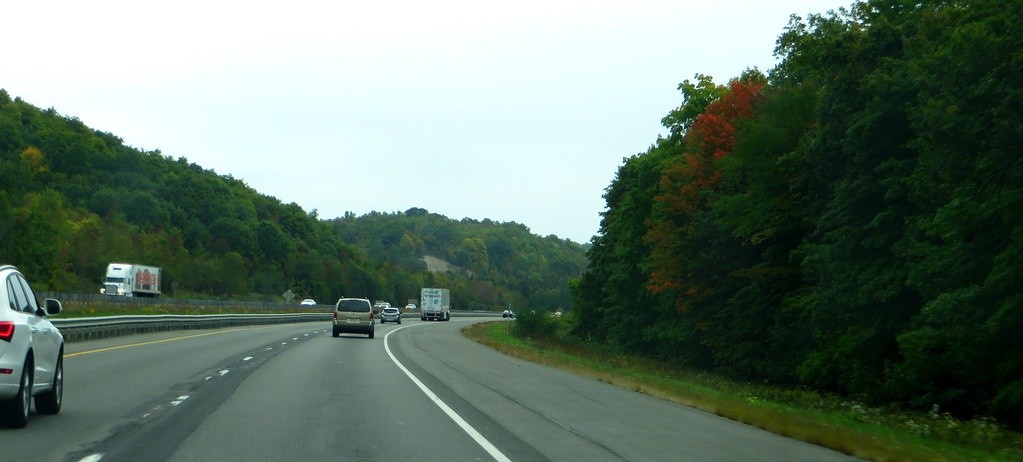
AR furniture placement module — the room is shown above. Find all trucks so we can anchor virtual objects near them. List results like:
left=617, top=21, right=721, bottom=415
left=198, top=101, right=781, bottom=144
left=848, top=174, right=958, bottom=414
left=100, top=263, right=161, bottom=299
left=421, top=288, right=452, bottom=321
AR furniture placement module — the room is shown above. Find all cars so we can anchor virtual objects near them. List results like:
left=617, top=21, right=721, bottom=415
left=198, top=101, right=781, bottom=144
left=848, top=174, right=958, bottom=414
left=503, top=310, right=514, bottom=318
left=333, top=296, right=378, bottom=339
left=381, top=308, right=401, bottom=324
left=0, top=264, right=65, bottom=428
left=301, top=299, right=317, bottom=306
left=555, top=312, right=562, bottom=316
left=373, top=302, right=391, bottom=309
left=405, top=304, right=416, bottom=309
